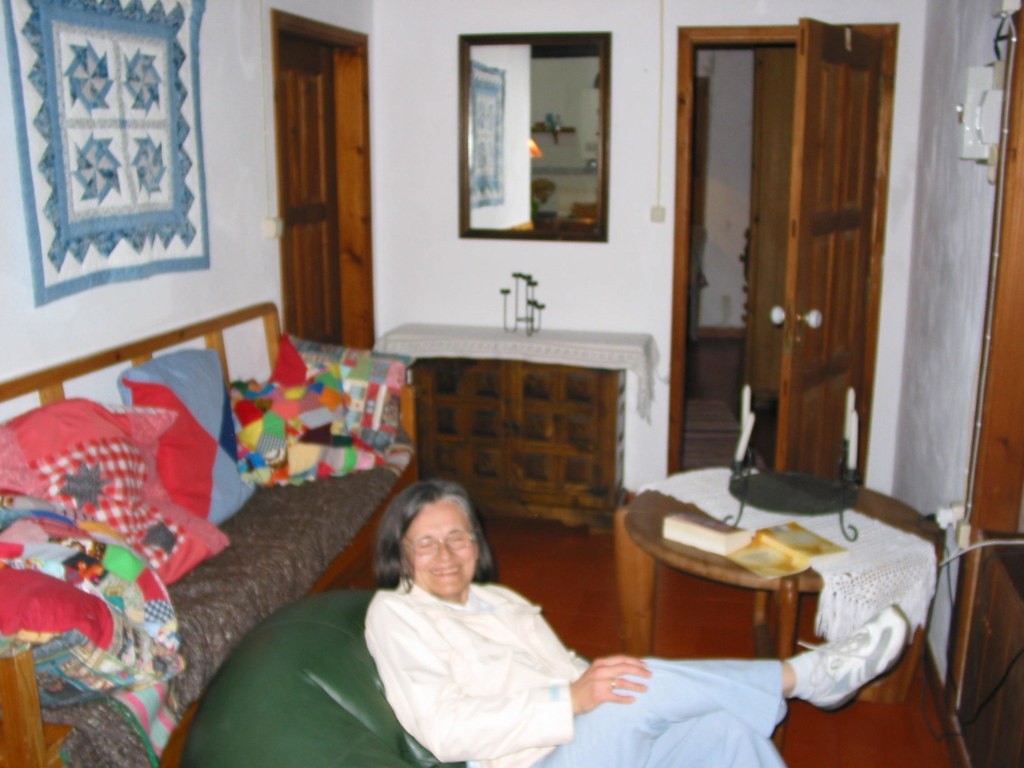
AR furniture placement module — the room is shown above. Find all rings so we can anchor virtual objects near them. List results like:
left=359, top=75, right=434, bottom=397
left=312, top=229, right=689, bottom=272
left=611, top=678, right=615, bottom=690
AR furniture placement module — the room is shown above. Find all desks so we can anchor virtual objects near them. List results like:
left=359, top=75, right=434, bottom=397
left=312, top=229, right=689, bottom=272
left=625, top=466, right=946, bottom=753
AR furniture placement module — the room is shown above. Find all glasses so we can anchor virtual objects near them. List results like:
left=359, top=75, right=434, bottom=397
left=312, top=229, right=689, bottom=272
left=403, top=530, right=481, bottom=554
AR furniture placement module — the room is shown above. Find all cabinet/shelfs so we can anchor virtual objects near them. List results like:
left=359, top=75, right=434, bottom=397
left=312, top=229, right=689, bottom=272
left=371, top=321, right=660, bottom=537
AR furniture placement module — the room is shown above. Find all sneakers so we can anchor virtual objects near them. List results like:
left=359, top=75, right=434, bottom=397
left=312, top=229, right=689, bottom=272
left=797, top=604, right=910, bottom=712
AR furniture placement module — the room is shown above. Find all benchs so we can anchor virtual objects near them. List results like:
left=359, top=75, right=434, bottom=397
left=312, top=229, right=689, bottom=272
left=0, top=301, right=417, bottom=768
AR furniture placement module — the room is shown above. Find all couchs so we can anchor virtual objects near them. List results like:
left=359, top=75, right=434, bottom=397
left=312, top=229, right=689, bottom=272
left=179, top=588, right=466, bottom=768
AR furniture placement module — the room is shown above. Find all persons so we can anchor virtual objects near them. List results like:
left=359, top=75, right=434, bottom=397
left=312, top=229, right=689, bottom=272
left=363, top=479, right=912, bottom=768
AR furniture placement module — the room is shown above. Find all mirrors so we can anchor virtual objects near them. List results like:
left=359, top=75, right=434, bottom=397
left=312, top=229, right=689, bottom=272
left=457, top=29, right=613, bottom=244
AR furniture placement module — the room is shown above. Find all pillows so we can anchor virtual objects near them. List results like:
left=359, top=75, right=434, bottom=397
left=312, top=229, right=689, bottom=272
left=0, top=561, right=188, bottom=710
left=268, top=332, right=417, bottom=461
left=116, top=347, right=255, bottom=525
left=0, top=397, right=230, bottom=584
left=227, top=361, right=385, bottom=487
left=0, top=488, right=182, bottom=652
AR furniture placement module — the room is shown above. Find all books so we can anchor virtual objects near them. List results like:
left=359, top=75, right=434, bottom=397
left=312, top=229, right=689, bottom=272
left=662, top=510, right=750, bottom=556
left=725, top=520, right=850, bottom=579
